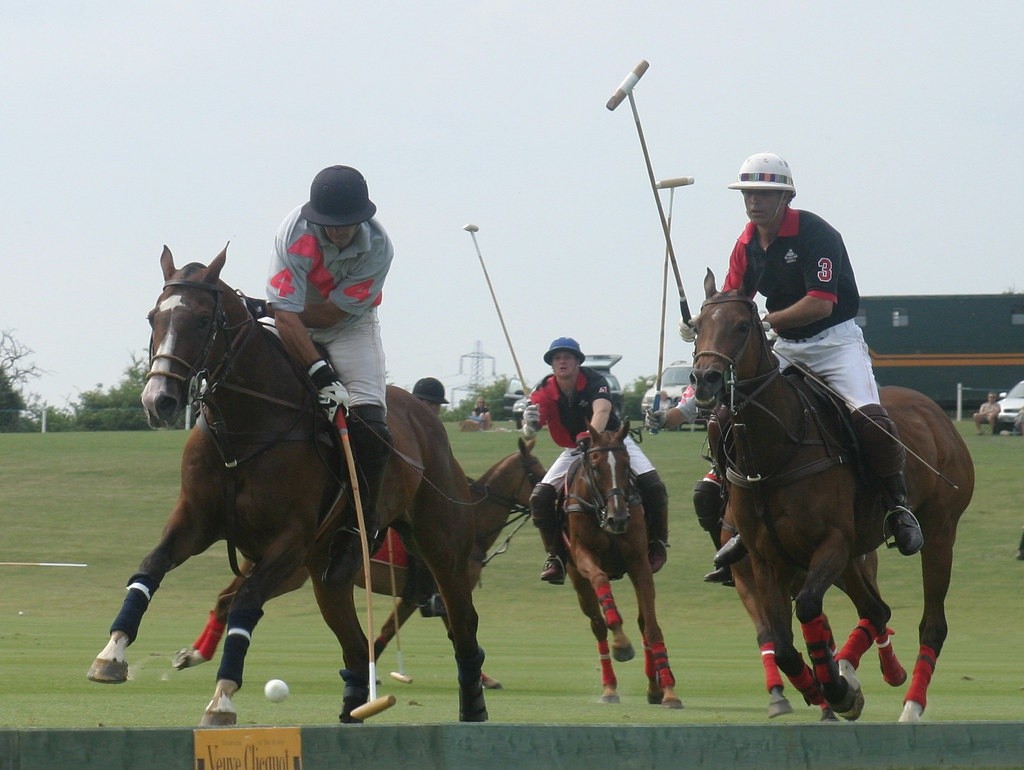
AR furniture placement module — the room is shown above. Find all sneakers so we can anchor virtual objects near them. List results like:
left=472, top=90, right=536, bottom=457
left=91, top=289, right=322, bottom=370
left=540, top=558, right=566, bottom=581
left=649, top=545, right=668, bottom=574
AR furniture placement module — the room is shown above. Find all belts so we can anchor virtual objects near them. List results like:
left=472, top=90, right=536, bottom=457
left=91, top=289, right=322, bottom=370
left=781, top=333, right=829, bottom=344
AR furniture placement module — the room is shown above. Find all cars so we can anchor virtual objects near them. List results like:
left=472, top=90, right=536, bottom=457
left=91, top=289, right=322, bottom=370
left=986, top=380, right=1024, bottom=436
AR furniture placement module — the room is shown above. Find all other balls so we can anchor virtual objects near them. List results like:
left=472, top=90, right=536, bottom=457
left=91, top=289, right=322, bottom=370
left=265, top=679, right=289, bottom=704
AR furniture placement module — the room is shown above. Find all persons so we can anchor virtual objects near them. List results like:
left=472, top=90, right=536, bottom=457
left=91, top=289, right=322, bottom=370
left=522, top=337, right=668, bottom=585
left=677, top=154, right=924, bottom=565
left=659, top=390, right=676, bottom=411
left=973, top=391, right=1001, bottom=435
left=268, top=166, right=394, bottom=551
left=648, top=393, right=735, bottom=583
left=407, top=375, right=451, bottom=619
left=472, top=398, right=491, bottom=430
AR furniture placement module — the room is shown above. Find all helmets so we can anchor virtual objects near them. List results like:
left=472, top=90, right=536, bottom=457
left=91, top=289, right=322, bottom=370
left=410, top=377, right=450, bottom=404
left=543, top=337, right=585, bottom=366
left=728, top=153, right=796, bottom=193
left=301, top=166, right=377, bottom=226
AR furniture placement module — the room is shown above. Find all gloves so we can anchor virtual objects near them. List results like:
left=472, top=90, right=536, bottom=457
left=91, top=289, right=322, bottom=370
left=523, top=403, right=540, bottom=438
left=759, top=312, right=771, bottom=333
left=682, top=315, right=700, bottom=343
left=237, top=293, right=266, bottom=319
left=307, top=358, right=349, bottom=422
left=575, top=430, right=593, bottom=450
left=645, top=407, right=667, bottom=429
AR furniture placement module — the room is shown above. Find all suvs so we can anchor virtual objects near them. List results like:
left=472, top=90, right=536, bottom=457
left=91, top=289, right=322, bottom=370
left=639, top=358, right=711, bottom=432
left=502, top=353, right=626, bottom=430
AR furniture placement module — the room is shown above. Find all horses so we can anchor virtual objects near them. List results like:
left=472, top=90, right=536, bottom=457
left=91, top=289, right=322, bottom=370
left=88, top=241, right=489, bottom=722
left=173, top=438, right=548, bottom=690
left=691, top=266, right=975, bottom=724
left=558, top=416, right=685, bottom=711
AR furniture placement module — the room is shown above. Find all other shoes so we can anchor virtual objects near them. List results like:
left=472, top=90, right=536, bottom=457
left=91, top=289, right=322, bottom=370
left=322, top=511, right=381, bottom=586
left=889, top=508, right=923, bottom=555
left=714, top=533, right=744, bottom=566
left=407, top=591, right=445, bottom=613
left=703, top=565, right=733, bottom=584
